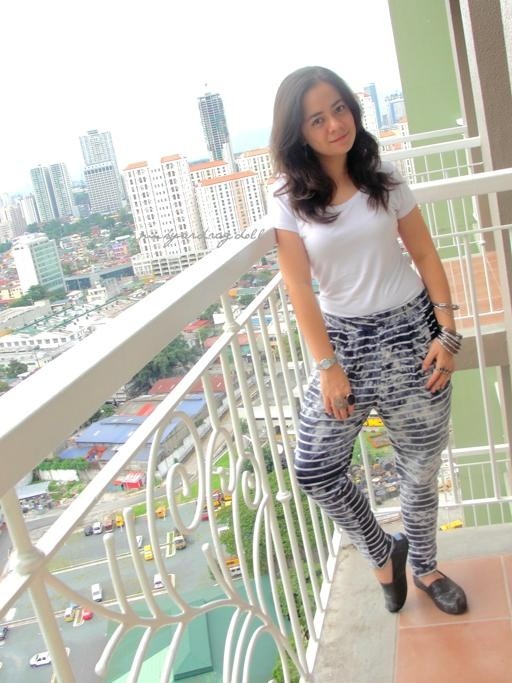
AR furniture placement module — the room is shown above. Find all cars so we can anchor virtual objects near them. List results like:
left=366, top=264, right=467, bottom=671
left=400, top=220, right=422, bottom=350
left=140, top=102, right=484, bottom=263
left=63, top=601, right=93, bottom=622
left=173, top=529, right=186, bottom=550
left=29, top=648, right=70, bottom=667
left=154, top=574, right=164, bottom=588
left=0, top=626, right=9, bottom=640
left=135, top=535, right=153, bottom=561
left=90, top=583, right=102, bottom=603
left=201, top=489, right=233, bottom=523
left=84, top=516, right=125, bottom=537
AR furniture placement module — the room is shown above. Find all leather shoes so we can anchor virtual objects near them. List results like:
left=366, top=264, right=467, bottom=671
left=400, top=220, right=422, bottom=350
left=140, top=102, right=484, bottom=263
left=380, top=532, right=408, bottom=612
left=414, top=569, right=466, bottom=614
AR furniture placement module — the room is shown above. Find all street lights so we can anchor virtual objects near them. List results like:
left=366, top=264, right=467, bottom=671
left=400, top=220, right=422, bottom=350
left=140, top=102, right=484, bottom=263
left=31, top=344, right=44, bottom=368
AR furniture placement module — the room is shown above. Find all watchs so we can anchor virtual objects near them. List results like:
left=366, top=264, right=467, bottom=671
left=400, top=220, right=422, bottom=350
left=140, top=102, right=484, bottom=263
left=313, top=355, right=338, bottom=370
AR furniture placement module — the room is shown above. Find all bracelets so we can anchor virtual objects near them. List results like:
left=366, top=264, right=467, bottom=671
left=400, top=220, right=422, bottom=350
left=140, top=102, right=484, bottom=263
left=431, top=300, right=462, bottom=356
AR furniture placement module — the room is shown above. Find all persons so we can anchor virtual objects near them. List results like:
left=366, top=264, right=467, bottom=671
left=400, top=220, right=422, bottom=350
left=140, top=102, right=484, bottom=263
left=263, top=65, right=471, bottom=616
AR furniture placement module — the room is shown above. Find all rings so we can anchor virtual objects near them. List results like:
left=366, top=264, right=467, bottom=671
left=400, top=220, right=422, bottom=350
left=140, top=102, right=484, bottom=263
left=333, top=399, right=344, bottom=411
left=345, top=392, right=355, bottom=405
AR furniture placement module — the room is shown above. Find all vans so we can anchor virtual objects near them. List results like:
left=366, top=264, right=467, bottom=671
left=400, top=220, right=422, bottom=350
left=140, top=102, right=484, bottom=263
left=442, top=521, right=462, bottom=531
